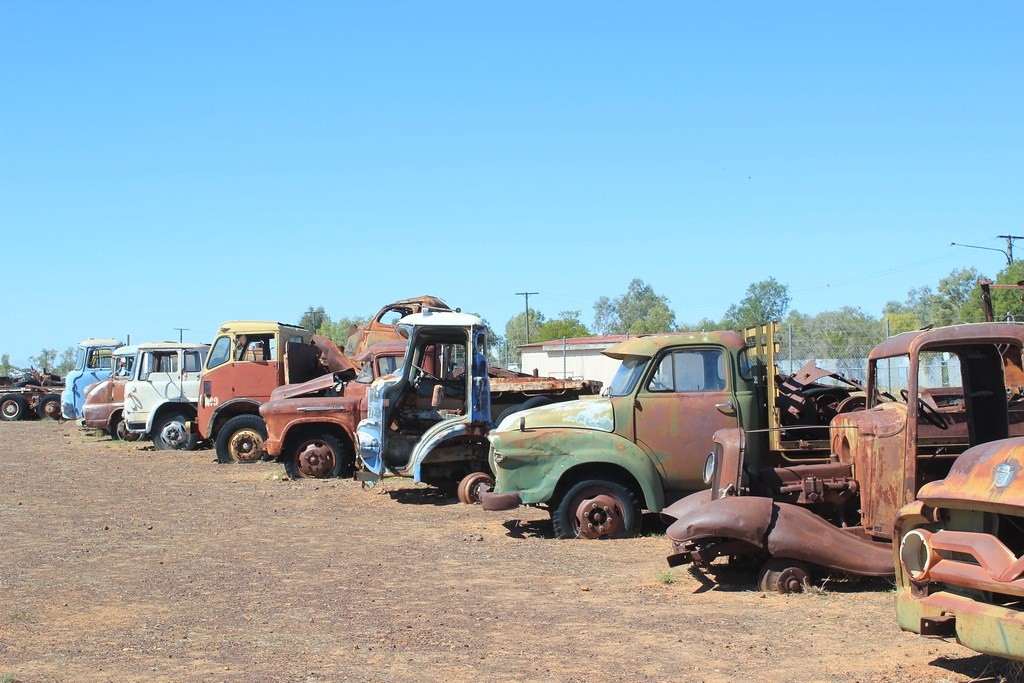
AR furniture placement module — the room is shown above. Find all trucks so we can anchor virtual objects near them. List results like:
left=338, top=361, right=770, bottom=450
left=486, top=322, right=1024, bottom=541
left=124, top=343, right=212, bottom=453
left=890, top=431, right=1023, bottom=666
left=346, top=295, right=602, bottom=400
left=665, top=320, right=1023, bottom=597
left=345, top=343, right=448, bottom=423
left=196, top=319, right=359, bottom=484
left=357, top=308, right=495, bottom=506
left=0, top=370, right=63, bottom=420
left=60, top=337, right=126, bottom=422
left=81, top=343, right=144, bottom=443
